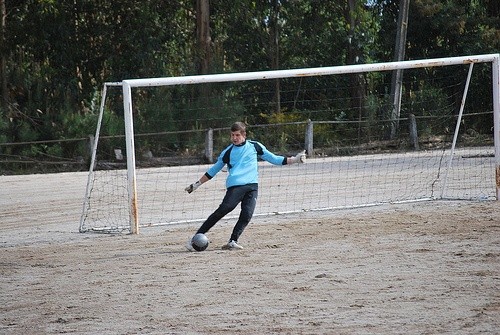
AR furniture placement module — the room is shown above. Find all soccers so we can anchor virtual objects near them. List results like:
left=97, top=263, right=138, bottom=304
left=191, top=233, right=209, bottom=252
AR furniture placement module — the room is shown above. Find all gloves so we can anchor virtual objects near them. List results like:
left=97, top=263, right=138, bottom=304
left=291, top=149, right=306, bottom=164
left=185, top=180, right=201, bottom=194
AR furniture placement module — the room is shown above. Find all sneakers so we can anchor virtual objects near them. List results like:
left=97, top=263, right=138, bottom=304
left=228, top=239, right=243, bottom=249
left=187, top=242, right=195, bottom=251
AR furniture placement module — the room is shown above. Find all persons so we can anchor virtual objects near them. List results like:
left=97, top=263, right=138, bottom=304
left=185, top=123, right=306, bottom=251
